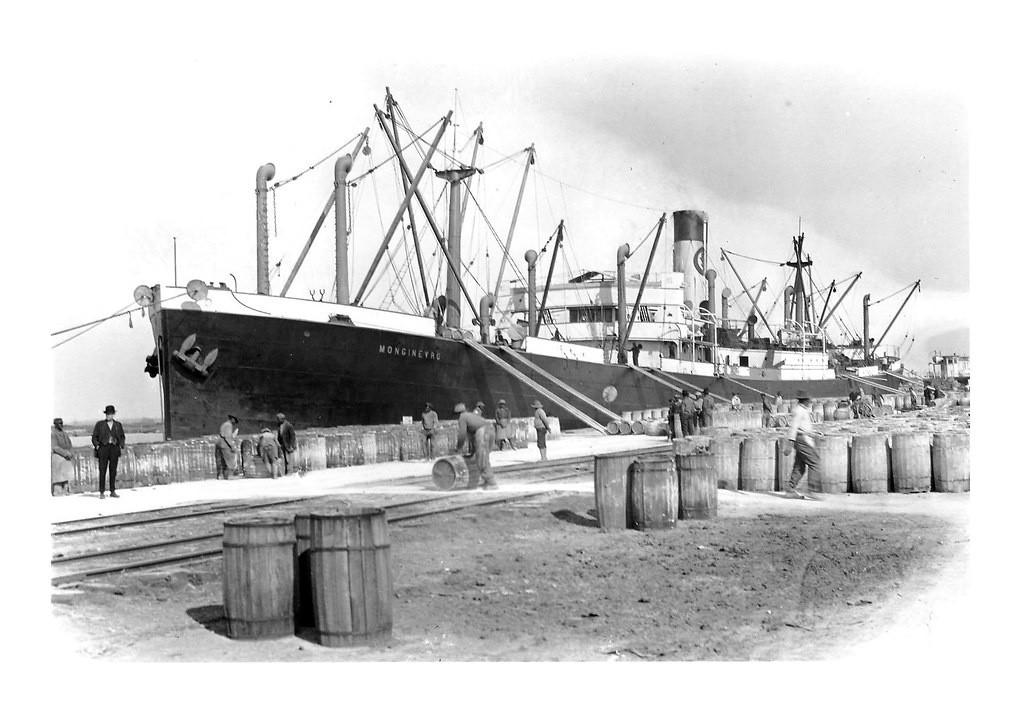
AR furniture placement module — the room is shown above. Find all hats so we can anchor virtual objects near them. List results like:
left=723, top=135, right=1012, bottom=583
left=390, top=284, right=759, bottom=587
left=424, top=401, right=433, bottom=410
left=453, top=401, right=466, bottom=413
left=530, top=399, right=544, bottom=409
left=796, top=389, right=811, bottom=401
left=261, top=428, right=271, bottom=433
left=498, top=397, right=507, bottom=406
left=227, top=413, right=240, bottom=424
left=666, top=387, right=712, bottom=403
left=102, top=405, right=120, bottom=414
left=276, top=413, right=286, bottom=419
left=477, top=401, right=485, bottom=408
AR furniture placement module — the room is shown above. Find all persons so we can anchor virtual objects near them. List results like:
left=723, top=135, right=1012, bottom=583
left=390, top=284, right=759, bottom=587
left=51, top=418, right=74, bottom=496
left=276, top=413, right=296, bottom=476
left=849, top=385, right=864, bottom=403
left=776, top=391, right=783, bottom=406
left=783, top=390, right=827, bottom=501
left=668, top=387, right=714, bottom=442
left=215, top=415, right=239, bottom=480
left=731, top=392, right=740, bottom=409
left=92, top=406, right=125, bottom=499
left=421, top=402, right=439, bottom=463
left=257, top=428, right=281, bottom=479
left=909, top=387, right=917, bottom=410
left=933, top=384, right=944, bottom=399
left=495, top=399, right=518, bottom=451
left=761, top=393, right=772, bottom=428
left=468, top=402, right=485, bottom=453
left=627, top=343, right=640, bottom=366
left=454, top=402, right=498, bottom=490
left=531, top=400, right=552, bottom=462
left=924, top=387, right=932, bottom=405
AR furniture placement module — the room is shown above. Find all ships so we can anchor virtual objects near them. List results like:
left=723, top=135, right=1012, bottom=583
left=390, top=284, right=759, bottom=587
left=135, top=89, right=927, bottom=440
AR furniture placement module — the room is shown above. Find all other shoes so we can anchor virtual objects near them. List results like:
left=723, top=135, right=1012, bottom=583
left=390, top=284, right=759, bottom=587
left=810, top=491, right=828, bottom=502
left=784, top=489, right=805, bottom=499
left=101, top=493, right=106, bottom=500
left=110, top=491, right=121, bottom=499
left=483, top=482, right=501, bottom=490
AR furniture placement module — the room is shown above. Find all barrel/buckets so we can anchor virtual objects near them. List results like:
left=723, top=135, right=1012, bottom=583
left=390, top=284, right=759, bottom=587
left=69, top=416, right=532, bottom=493
left=221, top=499, right=395, bottom=647
left=594, top=385, right=971, bottom=533
left=438, top=327, right=475, bottom=341
